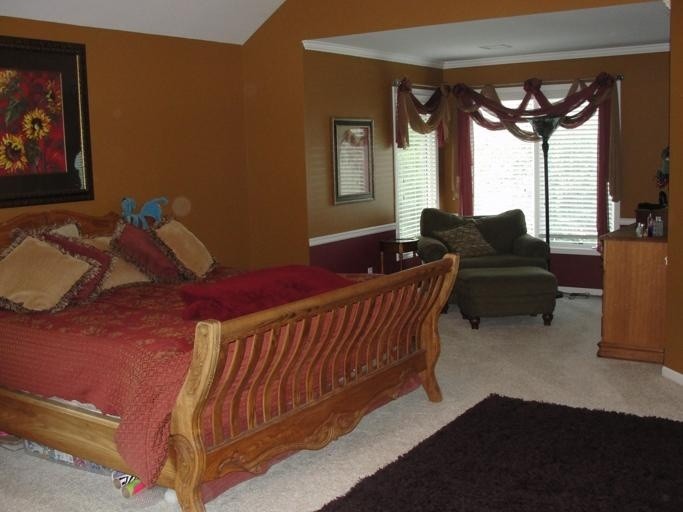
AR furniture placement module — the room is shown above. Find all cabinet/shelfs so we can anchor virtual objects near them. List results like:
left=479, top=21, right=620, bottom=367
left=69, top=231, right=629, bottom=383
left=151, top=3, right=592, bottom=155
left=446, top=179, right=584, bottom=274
left=594, top=221, right=672, bottom=365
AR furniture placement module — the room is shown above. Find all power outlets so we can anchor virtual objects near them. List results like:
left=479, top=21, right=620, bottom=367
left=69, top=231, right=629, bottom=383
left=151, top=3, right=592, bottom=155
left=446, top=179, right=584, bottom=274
left=367, top=265, right=373, bottom=273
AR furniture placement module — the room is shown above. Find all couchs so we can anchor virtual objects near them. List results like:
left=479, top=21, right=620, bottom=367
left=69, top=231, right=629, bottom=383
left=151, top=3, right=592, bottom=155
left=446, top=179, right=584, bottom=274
left=453, top=262, right=559, bottom=328
left=411, top=202, right=551, bottom=315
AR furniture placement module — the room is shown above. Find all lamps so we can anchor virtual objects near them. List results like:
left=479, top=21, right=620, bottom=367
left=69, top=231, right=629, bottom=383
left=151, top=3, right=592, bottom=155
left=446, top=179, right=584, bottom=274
left=527, top=113, right=567, bottom=300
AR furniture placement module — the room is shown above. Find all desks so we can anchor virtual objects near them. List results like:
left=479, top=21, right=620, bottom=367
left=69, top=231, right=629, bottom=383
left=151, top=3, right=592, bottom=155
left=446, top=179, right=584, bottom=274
left=376, top=232, right=428, bottom=277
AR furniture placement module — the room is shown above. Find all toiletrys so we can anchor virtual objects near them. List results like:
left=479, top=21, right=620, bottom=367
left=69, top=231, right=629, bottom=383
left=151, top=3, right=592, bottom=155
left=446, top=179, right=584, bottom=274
left=634, top=211, right=665, bottom=239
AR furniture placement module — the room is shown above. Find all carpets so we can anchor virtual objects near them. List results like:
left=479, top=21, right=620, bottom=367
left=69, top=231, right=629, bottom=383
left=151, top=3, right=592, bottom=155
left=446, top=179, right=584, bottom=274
left=309, top=392, right=682, bottom=512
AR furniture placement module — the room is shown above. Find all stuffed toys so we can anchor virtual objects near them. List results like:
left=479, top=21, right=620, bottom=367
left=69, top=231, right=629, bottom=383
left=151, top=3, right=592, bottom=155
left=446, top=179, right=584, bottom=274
left=120, top=195, right=169, bottom=231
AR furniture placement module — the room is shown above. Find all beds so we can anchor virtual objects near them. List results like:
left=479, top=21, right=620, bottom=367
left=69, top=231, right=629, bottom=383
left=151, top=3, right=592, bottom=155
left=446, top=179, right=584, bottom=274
left=0, top=205, right=459, bottom=512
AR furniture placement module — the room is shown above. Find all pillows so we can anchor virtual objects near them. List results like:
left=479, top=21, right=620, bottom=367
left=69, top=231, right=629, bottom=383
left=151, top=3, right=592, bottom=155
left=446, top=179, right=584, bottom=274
left=72, top=235, right=156, bottom=296
left=42, top=234, right=120, bottom=310
left=0, top=228, right=96, bottom=314
left=101, top=220, right=176, bottom=283
left=146, top=213, right=215, bottom=280
left=430, top=218, right=495, bottom=258
left=36, top=217, right=78, bottom=233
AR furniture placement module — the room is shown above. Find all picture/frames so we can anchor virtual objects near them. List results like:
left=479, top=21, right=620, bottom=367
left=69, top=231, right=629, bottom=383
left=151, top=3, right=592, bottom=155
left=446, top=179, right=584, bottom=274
left=0, top=33, right=96, bottom=213
left=326, top=114, right=377, bottom=206
